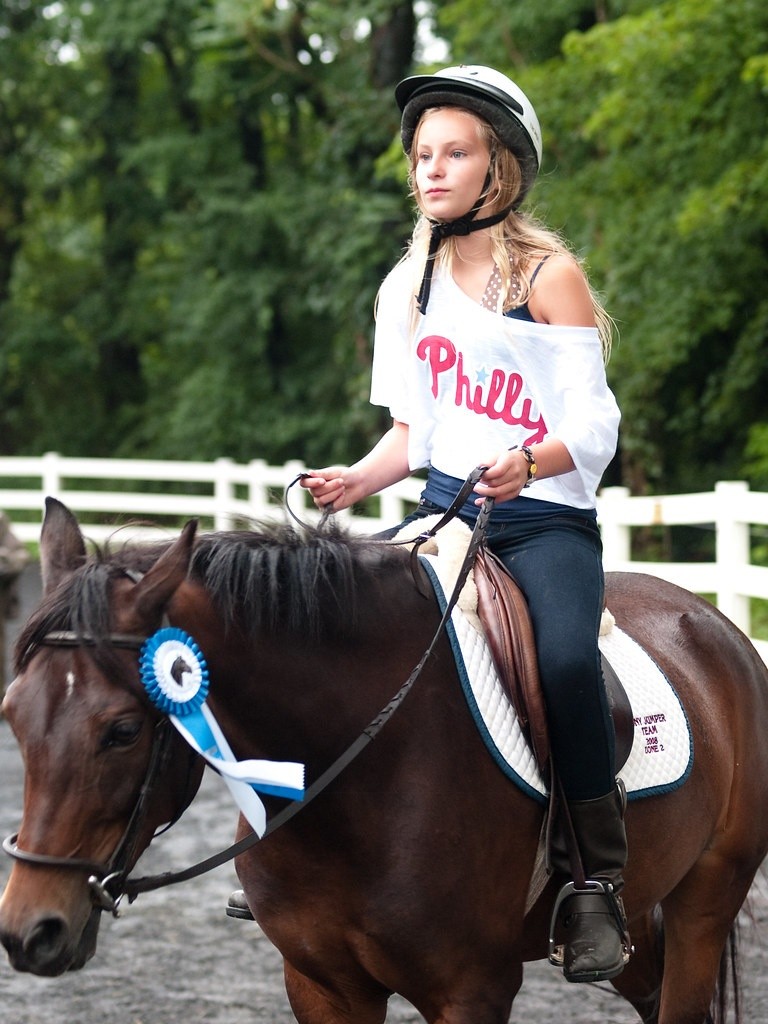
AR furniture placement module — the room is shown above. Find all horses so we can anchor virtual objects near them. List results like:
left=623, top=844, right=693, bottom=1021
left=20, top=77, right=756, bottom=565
left=1, top=497, right=768, bottom=1024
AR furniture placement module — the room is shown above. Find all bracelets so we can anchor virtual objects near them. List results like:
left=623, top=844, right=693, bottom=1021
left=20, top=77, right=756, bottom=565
left=507, top=443, right=538, bottom=489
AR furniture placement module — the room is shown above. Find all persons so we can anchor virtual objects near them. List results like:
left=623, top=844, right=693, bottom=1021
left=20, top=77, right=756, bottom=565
left=226, top=64, right=636, bottom=984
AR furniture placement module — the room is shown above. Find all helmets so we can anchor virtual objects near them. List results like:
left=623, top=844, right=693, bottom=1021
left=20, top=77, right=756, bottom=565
left=394, top=64, right=543, bottom=212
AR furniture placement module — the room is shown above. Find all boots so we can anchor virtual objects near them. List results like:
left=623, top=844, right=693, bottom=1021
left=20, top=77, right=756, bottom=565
left=227, top=890, right=255, bottom=920
left=563, top=786, right=634, bottom=983
